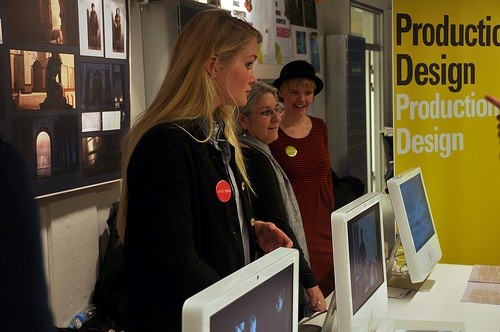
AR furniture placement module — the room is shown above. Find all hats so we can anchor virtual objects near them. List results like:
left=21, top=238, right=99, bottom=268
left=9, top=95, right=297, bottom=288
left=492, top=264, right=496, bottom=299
left=272, top=59, right=324, bottom=102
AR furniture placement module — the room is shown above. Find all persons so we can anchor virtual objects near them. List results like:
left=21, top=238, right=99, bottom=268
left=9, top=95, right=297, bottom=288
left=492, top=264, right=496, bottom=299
left=236, top=80, right=327, bottom=322
left=485, top=94, right=500, bottom=137
left=114, top=9, right=293, bottom=332
left=268, top=59, right=335, bottom=299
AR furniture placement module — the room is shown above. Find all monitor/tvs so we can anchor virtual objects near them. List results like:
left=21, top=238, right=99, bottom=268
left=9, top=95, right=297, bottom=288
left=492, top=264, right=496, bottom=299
left=321, top=192, right=389, bottom=332
left=387, top=166, right=442, bottom=291
left=181, top=246, right=299, bottom=332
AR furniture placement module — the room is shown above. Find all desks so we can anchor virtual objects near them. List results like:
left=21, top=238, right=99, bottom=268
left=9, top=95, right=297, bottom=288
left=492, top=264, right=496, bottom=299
left=298, top=263, right=500, bottom=332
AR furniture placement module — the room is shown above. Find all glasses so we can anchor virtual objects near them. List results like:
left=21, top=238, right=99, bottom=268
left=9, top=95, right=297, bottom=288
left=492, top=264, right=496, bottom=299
left=244, top=106, right=285, bottom=117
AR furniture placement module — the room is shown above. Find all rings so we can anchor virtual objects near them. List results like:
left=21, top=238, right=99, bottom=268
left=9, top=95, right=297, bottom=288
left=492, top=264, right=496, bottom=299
left=318, top=300, right=321, bottom=305
